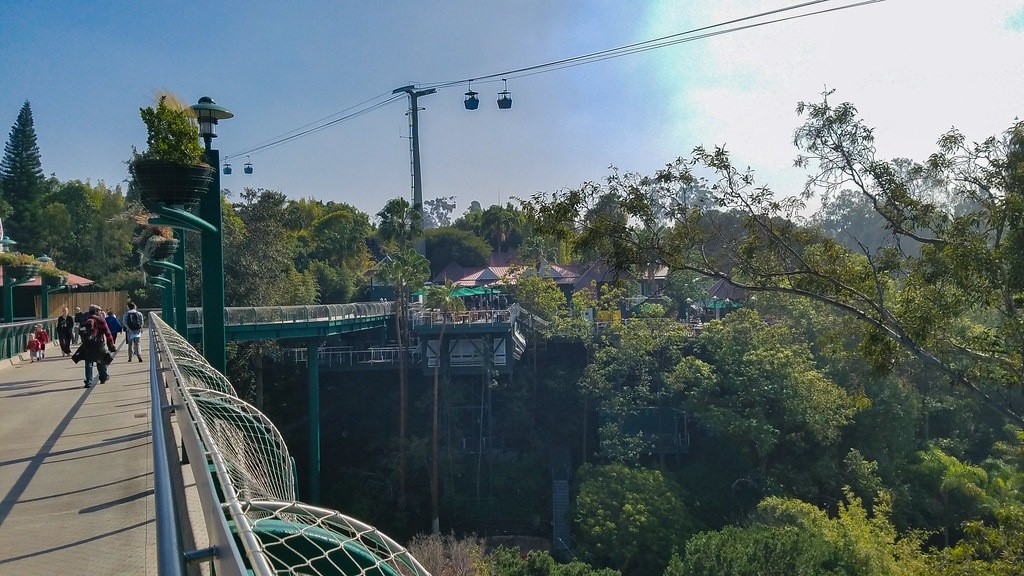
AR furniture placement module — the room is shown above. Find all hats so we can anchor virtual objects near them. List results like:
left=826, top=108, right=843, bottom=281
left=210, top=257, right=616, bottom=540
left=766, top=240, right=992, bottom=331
left=89, top=303, right=103, bottom=310
left=34, top=323, right=43, bottom=328
left=75, top=306, right=81, bottom=310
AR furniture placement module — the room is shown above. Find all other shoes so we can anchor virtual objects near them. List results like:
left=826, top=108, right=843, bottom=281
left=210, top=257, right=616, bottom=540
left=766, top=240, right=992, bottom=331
left=37, top=358, right=39, bottom=361
left=138, top=356, right=143, bottom=362
left=128, top=359, right=132, bottom=362
left=84, top=384, right=91, bottom=387
left=101, top=375, right=110, bottom=384
left=31, top=361, right=34, bottom=363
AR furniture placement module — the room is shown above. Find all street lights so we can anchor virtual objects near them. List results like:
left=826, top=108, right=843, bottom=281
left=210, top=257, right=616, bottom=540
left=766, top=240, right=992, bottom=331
left=712, top=295, right=720, bottom=320
left=724, top=298, right=730, bottom=314
left=36, top=253, right=68, bottom=319
left=0, top=235, right=52, bottom=323
left=684, top=298, right=693, bottom=323
left=128, top=96, right=235, bottom=376
left=750, top=296, right=756, bottom=310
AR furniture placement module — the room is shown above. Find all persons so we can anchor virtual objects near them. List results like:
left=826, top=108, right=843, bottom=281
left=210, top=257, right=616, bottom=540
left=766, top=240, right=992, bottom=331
left=35, top=323, right=49, bottom=359
left=82, top=301, right=144, bottom=363
left=26, top=333, right=41, bottom=362
left=74, top=306, right=84, bottom=344
left=58, top=307, right=73, bottom=357
left=82, top=305, right=116, bottom=387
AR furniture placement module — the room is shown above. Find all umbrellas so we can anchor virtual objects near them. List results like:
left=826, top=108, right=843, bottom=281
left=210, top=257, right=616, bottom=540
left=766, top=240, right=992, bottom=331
left=410, top=286, right=501, bottom=310
left=707, top=300, right=744, bottom=308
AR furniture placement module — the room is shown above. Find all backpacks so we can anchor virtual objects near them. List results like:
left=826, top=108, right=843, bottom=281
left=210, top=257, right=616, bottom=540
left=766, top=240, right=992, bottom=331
left=79, top=315, right=99, bottom=345
left=127, top=312, right=141, bottom=330
